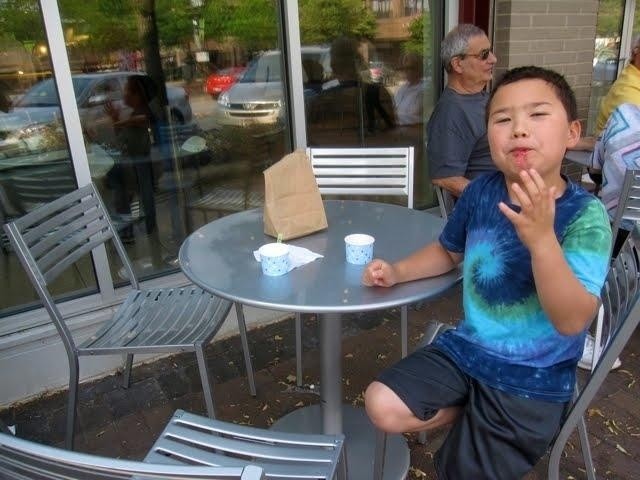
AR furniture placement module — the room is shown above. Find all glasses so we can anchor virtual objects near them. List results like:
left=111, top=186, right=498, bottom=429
left=448, top=46, right=493, bottom=69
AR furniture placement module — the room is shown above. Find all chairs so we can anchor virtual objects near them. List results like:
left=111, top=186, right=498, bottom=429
left=418, top=225, right=640, bottom=480
left=295, top=146, right=415, bottom=388
left=414, top=138, right=457, bottom=312
left=3, top=184, right=256, bottom=456
left=612, top=167, right=640, bottom=257
left=0, top=409, right=347, bottom=480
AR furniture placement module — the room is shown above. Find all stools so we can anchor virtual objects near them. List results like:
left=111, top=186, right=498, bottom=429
left=564, top=136, right=598, bottom=169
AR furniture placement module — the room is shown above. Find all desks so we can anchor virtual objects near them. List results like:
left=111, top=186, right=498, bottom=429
left=178, top=200, right=464, bottom=479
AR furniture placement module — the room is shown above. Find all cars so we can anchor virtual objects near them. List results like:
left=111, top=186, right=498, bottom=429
left=368, top=59, right=398, bottom=87
left=203, top=66, right=248, bottom=97
left=1, top=74, right=193, bottom=142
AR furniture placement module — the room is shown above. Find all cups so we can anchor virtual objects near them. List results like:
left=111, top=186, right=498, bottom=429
left=258, top=243, right=290, bottom=277
left=344, top=233, right=375, bottom=265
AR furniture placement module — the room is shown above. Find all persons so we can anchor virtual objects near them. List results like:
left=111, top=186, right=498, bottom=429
left=582, top=104, right=639, bottom=268
left=390, top=50, right=431, bottom=127
left=424, top=23, right=621, bottom=372
left=591, top=36, right=640, bottom=140
left=83, top=74, right=165, bottom=245
left=307, top=36, right=398, bottom=149
left=361, top=64, right=613, bottom=479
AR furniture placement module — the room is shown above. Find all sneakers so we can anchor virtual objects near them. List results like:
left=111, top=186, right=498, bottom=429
left=577, top=334, right=621, bottom=371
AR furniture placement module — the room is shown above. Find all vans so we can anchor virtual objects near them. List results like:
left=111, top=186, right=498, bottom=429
left=219, top=48, right=370, bottom=130
